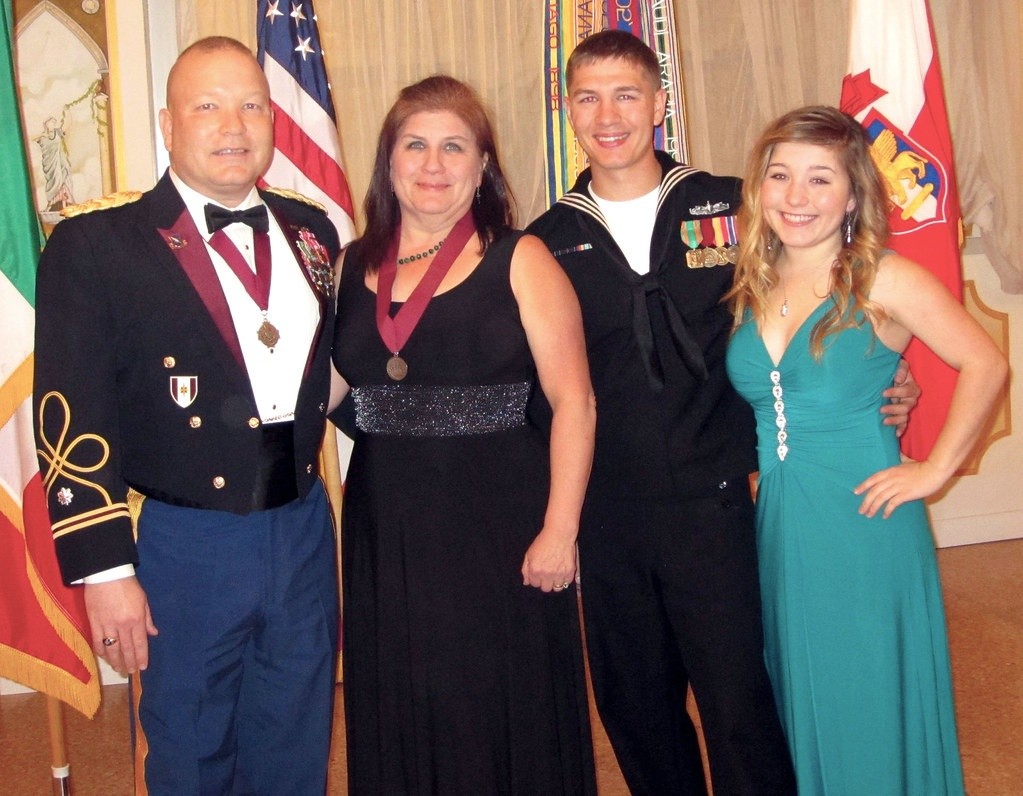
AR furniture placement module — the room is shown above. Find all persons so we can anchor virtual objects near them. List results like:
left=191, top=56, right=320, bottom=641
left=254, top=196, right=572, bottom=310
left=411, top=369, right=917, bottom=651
left=725, top=105, right=1010, bottom=796
left=326, top=74, right=598, bottom=796
left=31, top=36, right=340, bottom=796
left=521, top=27, right=923, bottom=796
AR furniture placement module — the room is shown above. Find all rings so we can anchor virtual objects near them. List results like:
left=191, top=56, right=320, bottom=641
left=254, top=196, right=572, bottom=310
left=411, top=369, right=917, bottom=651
left=102, top=638, right=119, bottom=645
left=553, top=584, right=564, bottom=589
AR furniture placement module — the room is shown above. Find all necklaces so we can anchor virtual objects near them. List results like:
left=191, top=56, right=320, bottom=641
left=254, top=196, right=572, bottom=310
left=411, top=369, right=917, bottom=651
left=398, top=236, right=444, bottom=266
left=779, top=272, right=790, bottom=318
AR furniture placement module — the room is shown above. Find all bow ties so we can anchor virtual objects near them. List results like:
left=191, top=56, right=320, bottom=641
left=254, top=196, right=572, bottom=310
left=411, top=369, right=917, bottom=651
left=205, top=203, right=270, bottom=234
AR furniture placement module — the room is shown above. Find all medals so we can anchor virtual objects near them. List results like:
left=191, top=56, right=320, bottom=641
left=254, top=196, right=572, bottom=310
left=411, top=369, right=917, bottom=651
left=386, top=353, right=408, bottom=380
left=258, top=317, right=280, bottom=347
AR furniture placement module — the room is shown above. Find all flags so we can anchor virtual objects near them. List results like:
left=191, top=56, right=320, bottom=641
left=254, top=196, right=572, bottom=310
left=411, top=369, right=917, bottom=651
left=0, top=0, right=104, bottom=720
left=841, top=0, right=963, bottom=467
left=257, top=0, right=361, bottom=682
